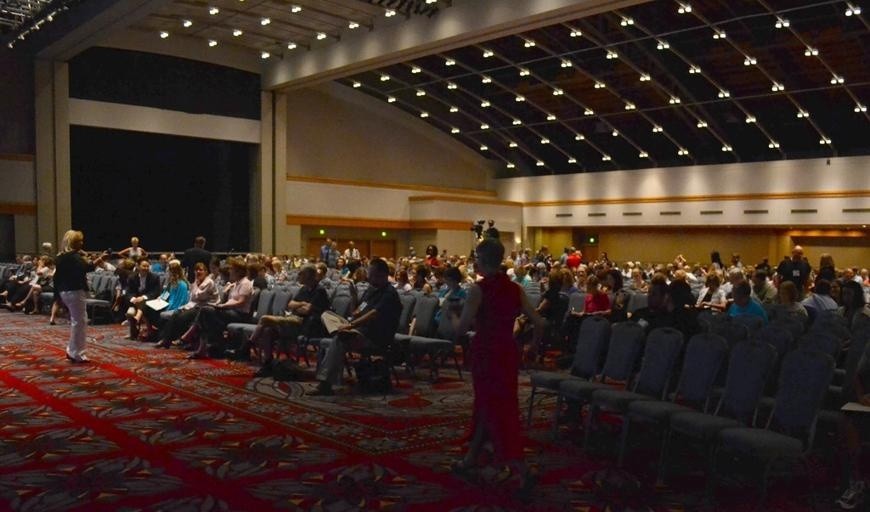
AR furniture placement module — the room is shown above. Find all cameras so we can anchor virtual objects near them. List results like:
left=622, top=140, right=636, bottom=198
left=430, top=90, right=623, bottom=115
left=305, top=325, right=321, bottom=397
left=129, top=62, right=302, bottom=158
left=470, top=220, right=485, bottom=239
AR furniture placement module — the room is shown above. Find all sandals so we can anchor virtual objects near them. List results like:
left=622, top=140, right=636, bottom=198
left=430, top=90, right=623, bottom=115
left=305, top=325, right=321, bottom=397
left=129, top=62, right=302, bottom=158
left=184, top=351, right=204, bottom=361
left=171, top=338, right=194, bottom=348
left=49, top=318, right=53, bottom=323
left=13, top=301, right=24, bottom=307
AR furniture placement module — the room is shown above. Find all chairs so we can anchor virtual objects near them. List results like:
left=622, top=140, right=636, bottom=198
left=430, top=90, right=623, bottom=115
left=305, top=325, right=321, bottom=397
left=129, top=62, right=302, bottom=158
left=618, top=335, right=726, bottom=475
left=393, top=299, right=438, bottom=377
left=409, top=300, right=468, bottom=383
left=323, top=303, right=402, bottom=384
left=303, top=293, right=355, bottom=377
left=524, top=282, right=650, bottom=312
left=668, top=342, right=780, bottom=491
left=223, top=289, right=275, bottom=356
left=277, top=279, right=441, bottom=296
left=245, top=289, right=293, bottom=359
left=551, top=321, right=645, bottom=441
left=717, top=349, right=835, bottom=512
left=85, top=272, right=124, bottom=321
left=587, top=328, right=686, bottom=464
left=526, top=318, right=608, bottom=432
left=660, top=307, right=870, bottom=354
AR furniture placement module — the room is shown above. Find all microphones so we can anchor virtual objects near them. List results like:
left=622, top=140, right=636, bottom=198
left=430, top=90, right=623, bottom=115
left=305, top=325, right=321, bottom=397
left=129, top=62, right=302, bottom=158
left=105, top=247, right=112, bottom=253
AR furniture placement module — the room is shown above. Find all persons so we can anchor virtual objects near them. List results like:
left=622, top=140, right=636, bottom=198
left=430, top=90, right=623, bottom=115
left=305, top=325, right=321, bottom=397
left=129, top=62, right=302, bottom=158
left=829, top=280, right=843, bottom=302
left=180, top=237, right=211, bottom=283
left=382, top=245, right=477, bottom=279
left=646, top=281, right=690, bottom=328
left=410, top=266, right=434, bottom=294
left=314, top=262, right=327, bottom=282
left=119, top=236, right=149, bottom=263
left=153, top=260, right=221, bottom=350
left=530, top=275, right=563, bottom=347
left=800, top=280, right=838, bottom=317
left=507, top=245, right=545, bottom=283
left=837, top=339, right=869, bottom=509
left=545, top=245, right=869, bottom=281
left=318, top=238, right=332, bottom=264
left=207, top=256, right=226, bottom=286
left=482, top=218, right=499, bottom=239
left=124, top=258, right=189, bottom=340
left=114, top=259, right=132, bottom=309
left=768, top=282, right=808, bottom=333
left=171, top=258, right=252, bottom=357
left=244, top=250, right=317, bottom=268
left=428, top=266, right=465, bottom=336
left=723, top=286, right=769, bottom=323
left=351, top=267, right=366, bottom=282
left=833, top=282, right=869, bottom=352
left=723, top=271, right=744, bottom=297
left=330, top=242, right=341, bottom=263
left=343, top=241, right=361, bottom=266
left=153, top=253, right=169, bottom=272
left=453, top=238, right=546, bottom=498
left=392, top=270, right=410, bottom=292
left=566, top=275, right=610, bottom=335
left=125, top=261, right=160, bottom=339
left=695, top=274, right=727, bottom=314
left=630, top=275, right=668, bottom=328
left=0, top=243, right=62, bottom=326
left=51, top=230, right=108, bottom=364
left=334, top=258, right=348, bottom=274
left=308, top=259, right=404, bottom=394
left=224, top=265, right=329, bottom=377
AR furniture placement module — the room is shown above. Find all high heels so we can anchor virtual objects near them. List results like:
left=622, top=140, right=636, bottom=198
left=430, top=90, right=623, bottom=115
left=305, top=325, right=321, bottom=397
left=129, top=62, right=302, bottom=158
left=507, top=473, right=529, bottom=491
left=448, top=464, right=478, bottom=483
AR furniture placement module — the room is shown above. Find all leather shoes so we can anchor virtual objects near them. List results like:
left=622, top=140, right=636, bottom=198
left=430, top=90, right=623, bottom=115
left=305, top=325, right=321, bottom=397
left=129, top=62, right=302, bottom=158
left=81, top=359, right=89, bottom=362
left=66, top=354, right=80, bottom=361
left=125, top=334, right=136, bottom=340
left=305, top=387, right=335, bottom=399
left=241, top=340, right=252, bottom=346
left=338, top=329, right=355, bottom=346
left=126, top=314, right=139, bottom=328
left=255, top=369, right=270, bottom=376
left=153, top=342, right=163, bottom=348
